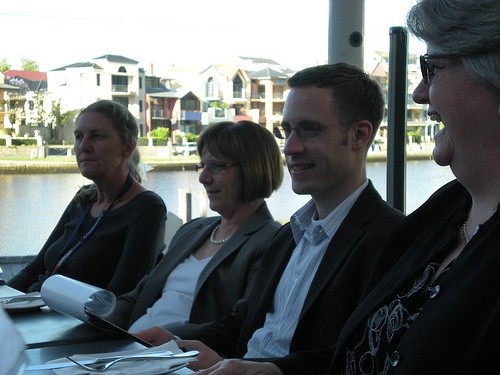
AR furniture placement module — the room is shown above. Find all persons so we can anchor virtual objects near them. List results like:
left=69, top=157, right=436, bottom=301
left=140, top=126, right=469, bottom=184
left=190, top=0, right=500, bottom=375
left=4, top=100, right=167, bottom=298
left=130, top=62, right=406, bottom=375
left=102, top=120, right=286, bottom=334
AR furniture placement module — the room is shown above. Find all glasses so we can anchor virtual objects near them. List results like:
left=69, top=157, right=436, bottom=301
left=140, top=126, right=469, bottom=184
left=420, top=53, right=468, bottom=84
left=195, top=160, right=239, bottom=174
left=273, top=120, right=359, bottom=139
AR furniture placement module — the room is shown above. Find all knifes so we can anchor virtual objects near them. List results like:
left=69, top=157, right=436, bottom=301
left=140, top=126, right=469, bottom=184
left=26, top=350, right=176, bottom=371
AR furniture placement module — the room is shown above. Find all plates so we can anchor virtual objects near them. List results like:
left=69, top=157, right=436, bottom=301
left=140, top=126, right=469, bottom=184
left=46, top=350, right=189, bottom=375
left=0, top=293, right=47, bottom=311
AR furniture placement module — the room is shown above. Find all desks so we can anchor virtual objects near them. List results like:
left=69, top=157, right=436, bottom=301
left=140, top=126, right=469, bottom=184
left=0, top=284, right=196, bottom=375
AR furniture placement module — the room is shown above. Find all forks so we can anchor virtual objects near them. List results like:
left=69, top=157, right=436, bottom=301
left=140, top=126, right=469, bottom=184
left=63, top=349, right=199, bottom=371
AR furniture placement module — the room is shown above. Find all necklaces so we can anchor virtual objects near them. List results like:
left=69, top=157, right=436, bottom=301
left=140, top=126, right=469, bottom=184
left=209, top=224, right=230, bottom=242
left=462, top=220, right=470, bottom=243
